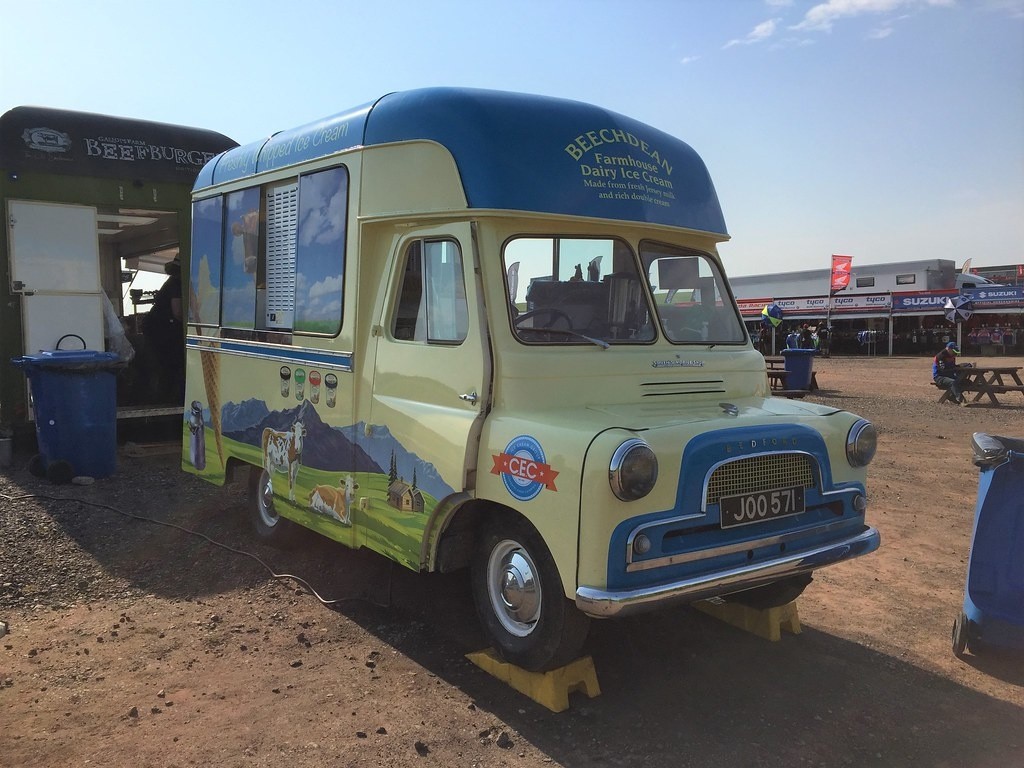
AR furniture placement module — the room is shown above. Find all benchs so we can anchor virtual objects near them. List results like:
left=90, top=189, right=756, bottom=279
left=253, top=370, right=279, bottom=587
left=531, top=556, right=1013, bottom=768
left=932, top=382, right=1024, bottom=395
left=765, top=368, right=810, bottom=400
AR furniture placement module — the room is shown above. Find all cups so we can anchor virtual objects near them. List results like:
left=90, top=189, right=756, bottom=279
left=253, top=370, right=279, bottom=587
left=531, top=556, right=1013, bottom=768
left=324, top=374, right=338, bottom=408
left=972, top=362, right=976, bottom=368
left=309, top=371, right=321, bottom=404
left=280, top=366, right=291, bottom=397
left=295, top=368, right=306, bottom=400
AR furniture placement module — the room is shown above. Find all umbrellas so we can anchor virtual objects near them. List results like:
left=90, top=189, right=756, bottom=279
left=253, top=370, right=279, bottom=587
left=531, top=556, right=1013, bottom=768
left=761, top=303, right=783, bottom=328
left=943, top=295, right=974, bottom=324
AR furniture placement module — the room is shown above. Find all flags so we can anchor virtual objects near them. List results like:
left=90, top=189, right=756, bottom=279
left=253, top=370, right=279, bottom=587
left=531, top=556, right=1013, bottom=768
left=830, top=254, right=852, bottom=290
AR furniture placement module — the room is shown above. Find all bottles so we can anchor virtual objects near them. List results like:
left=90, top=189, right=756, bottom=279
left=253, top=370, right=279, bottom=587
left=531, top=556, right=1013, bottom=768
left=190, top=401, right=205, bottom=470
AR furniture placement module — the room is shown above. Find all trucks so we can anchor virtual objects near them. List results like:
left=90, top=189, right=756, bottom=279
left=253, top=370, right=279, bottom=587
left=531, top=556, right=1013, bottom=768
left=179, top=87, right=880, bottom=673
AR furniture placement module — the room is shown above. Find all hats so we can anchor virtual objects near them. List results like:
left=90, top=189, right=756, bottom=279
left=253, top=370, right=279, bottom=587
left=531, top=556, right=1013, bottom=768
left=165, top=253, right=179, bottom=275
left=804, top=324, right=808, bottom=328
left=946, top=342, right=961, bottom=354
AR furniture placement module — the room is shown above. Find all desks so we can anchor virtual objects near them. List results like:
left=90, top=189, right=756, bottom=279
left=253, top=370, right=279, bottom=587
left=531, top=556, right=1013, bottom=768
left=765, top=359, right=785, bottom=386
left=936, top=367, right=1024, bottom=406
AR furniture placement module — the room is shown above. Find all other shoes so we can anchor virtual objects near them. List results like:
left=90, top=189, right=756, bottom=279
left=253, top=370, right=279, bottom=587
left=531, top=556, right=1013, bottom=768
left=956, top=394, right=967, bottom=404
left=948, top=397, right=958, bottom=405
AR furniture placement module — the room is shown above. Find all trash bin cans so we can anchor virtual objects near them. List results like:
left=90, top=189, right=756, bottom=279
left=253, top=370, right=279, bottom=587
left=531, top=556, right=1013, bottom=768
left=9, top=349, right=129, bottom=483
left=951, top=432, right=1024, bottom=657
left=780, top=348, right=819, bottom=390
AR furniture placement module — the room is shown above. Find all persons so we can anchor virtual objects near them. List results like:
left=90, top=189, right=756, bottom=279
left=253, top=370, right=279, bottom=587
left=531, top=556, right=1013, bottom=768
left=968, top=323, right=1024, bottom=345
left=932, top=342, right=972, bottom=406
left=758, top=322, right=765, bottom=356
left=141, top=253, right=186, bottom=404
left=786, top=322, right=830, bottom=358
